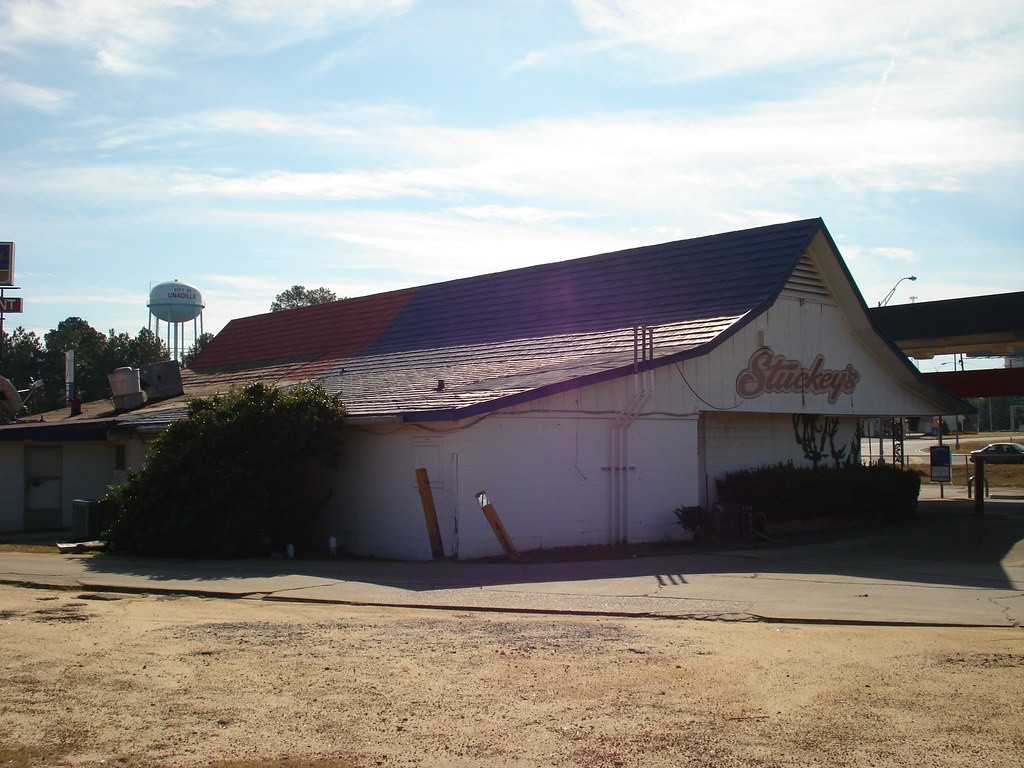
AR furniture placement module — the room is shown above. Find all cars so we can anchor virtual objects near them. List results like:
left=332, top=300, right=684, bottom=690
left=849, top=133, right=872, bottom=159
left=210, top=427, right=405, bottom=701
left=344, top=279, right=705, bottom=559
left=969, top=442, right=1024, bottom=464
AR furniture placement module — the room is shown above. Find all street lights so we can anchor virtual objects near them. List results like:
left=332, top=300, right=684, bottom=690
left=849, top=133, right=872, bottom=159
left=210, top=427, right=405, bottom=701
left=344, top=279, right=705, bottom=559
left=877, top=276, right=918, bottom=464
